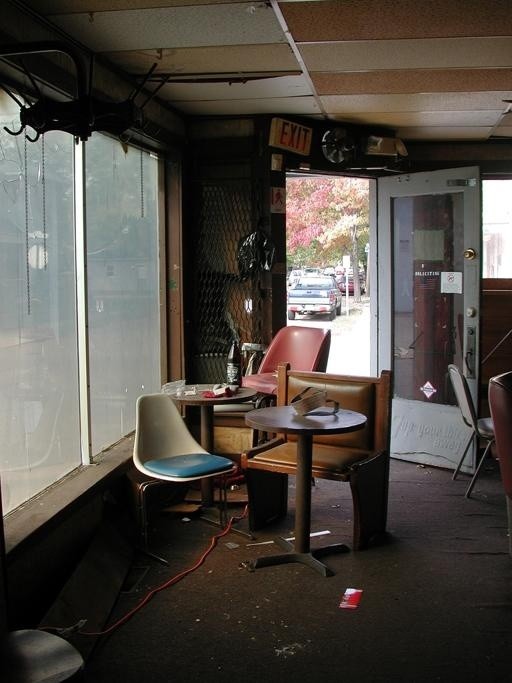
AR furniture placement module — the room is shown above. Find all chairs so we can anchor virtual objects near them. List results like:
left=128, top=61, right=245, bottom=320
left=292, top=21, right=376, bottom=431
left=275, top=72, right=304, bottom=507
left=487, top=371, right=512, bottom=537
left=133, top=393, right=253, bottom=565
left=242, top=326, right=331, bottom=406
left=241, top=362, right=393, bottom=551
left=448, top=364, right=495, bottom=498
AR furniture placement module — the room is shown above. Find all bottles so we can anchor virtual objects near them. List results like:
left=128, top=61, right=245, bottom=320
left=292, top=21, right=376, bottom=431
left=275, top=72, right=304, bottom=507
left=225, top=340, right=243, bottom=388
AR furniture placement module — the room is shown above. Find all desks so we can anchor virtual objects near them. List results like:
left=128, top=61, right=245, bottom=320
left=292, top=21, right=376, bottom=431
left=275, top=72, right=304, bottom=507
left=246, top=406, right=368, bottom=576
left=168, top=384, right=257, bottom=522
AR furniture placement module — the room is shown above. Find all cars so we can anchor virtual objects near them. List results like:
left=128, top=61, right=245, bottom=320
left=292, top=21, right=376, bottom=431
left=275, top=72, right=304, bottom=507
left=286, top=264, right=366, bottom=322
